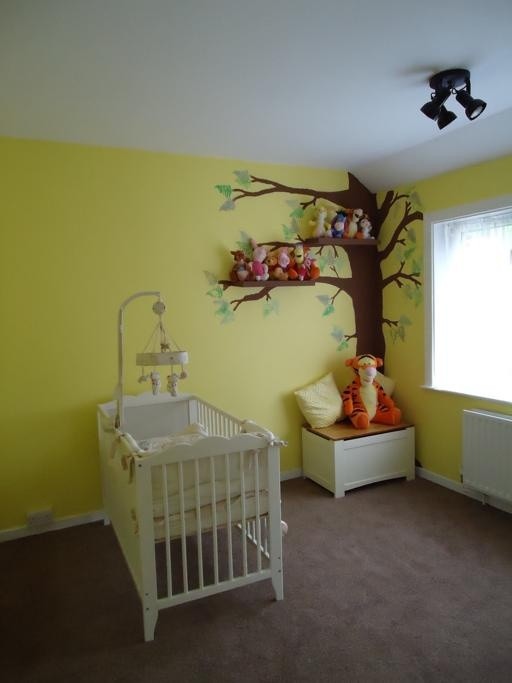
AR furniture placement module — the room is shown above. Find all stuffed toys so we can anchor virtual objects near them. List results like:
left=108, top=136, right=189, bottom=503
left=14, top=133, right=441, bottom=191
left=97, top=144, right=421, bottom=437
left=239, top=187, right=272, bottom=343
left=226, top=239, right=320, bottom=284
left=167, top=372, right=178, bottom=397
left=306, top=204, right=378, bottom=241
left=150, top=372, right=161, bottom=395
left=341, top=353, right=403, bottom=430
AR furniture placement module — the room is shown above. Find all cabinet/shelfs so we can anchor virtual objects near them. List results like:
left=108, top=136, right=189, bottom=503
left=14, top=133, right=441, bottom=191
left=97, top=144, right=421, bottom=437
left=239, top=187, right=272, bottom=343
left=217, top=236, right=381, bottom=290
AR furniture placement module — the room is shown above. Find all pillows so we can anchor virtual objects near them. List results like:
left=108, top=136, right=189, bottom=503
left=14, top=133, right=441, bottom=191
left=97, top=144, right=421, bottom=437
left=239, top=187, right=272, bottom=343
left=293, top=371, right=347, bottom=430
left=375, top=368, right=398, bottom=399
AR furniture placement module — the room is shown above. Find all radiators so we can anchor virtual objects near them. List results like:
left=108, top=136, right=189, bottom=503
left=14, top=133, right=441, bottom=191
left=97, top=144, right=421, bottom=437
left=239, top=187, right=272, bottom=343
left=459, top=407, right=512, bottom=506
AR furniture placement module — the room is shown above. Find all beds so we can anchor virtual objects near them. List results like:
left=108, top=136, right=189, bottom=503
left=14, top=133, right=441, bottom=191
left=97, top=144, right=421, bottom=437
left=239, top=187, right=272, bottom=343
left=97, top=390, right=287, bottom=642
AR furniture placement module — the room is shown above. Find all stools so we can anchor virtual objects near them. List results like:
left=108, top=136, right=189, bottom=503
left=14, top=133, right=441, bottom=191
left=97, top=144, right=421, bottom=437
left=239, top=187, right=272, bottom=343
left=299, top=417, right=419, bottom=498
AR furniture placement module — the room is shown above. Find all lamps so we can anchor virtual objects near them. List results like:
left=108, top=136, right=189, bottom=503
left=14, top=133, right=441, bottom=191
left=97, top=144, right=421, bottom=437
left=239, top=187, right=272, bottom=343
left=418, top=67, right=489, bottom=130
